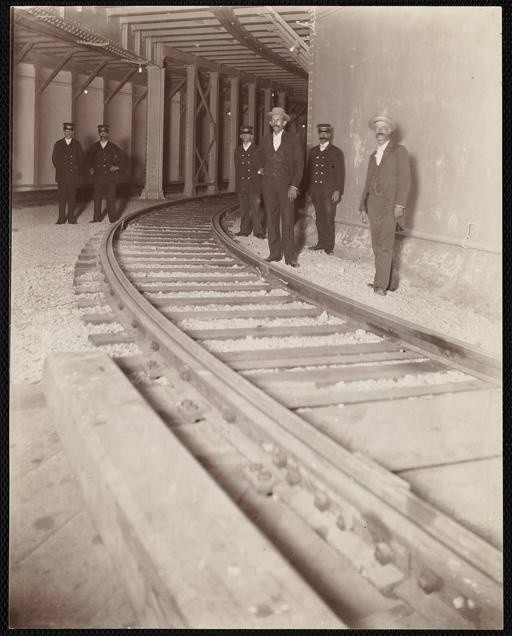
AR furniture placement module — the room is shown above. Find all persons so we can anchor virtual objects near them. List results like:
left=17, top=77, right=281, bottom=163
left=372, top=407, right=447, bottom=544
left=358, top=110, right=410, bottom=296
left=233, top=125, right=267, bottom=239
left=298, top=123, right=345, bottom=255
left=86, top=124, right=123, bottom=223
left=255, top=107, right=305, bottom=267
left=52, top=122, right=86, bottom=224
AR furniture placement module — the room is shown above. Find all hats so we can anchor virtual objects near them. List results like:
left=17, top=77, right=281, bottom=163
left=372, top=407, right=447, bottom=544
left=317, top=124, right=332, bottom=132
left=267, top=107, right=290, bottom=121
left=368, top=113, right=397, bottom=132
left=62, top=123, right=75, bottom=130
left=97, top=125, right=109, bottom=131
left=240, top=126, right=252, bottom=133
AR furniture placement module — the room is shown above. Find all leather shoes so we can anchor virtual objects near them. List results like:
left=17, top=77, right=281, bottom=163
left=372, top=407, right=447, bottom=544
left=367, top=282, right=388, bottom=296
left=264, top=257, right=299, bottom=267
left=235, top=232, right=265, bottom=239
left=56, top=219, right=115, bottom=225
left=309, top=244, right=334, bottom=255
